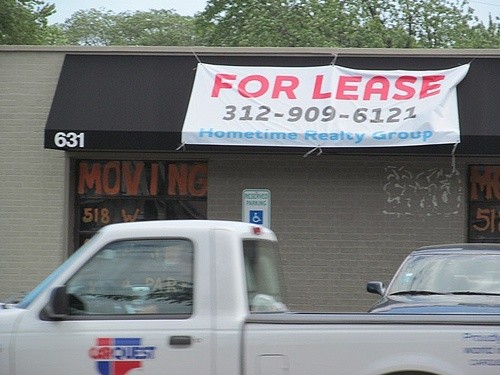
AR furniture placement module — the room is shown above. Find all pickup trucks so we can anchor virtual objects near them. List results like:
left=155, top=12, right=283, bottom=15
left=0, top=218, right=500, bottom=375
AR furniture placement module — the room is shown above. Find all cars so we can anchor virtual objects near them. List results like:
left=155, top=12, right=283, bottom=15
left=366, top=241, right=500, bottom=313
